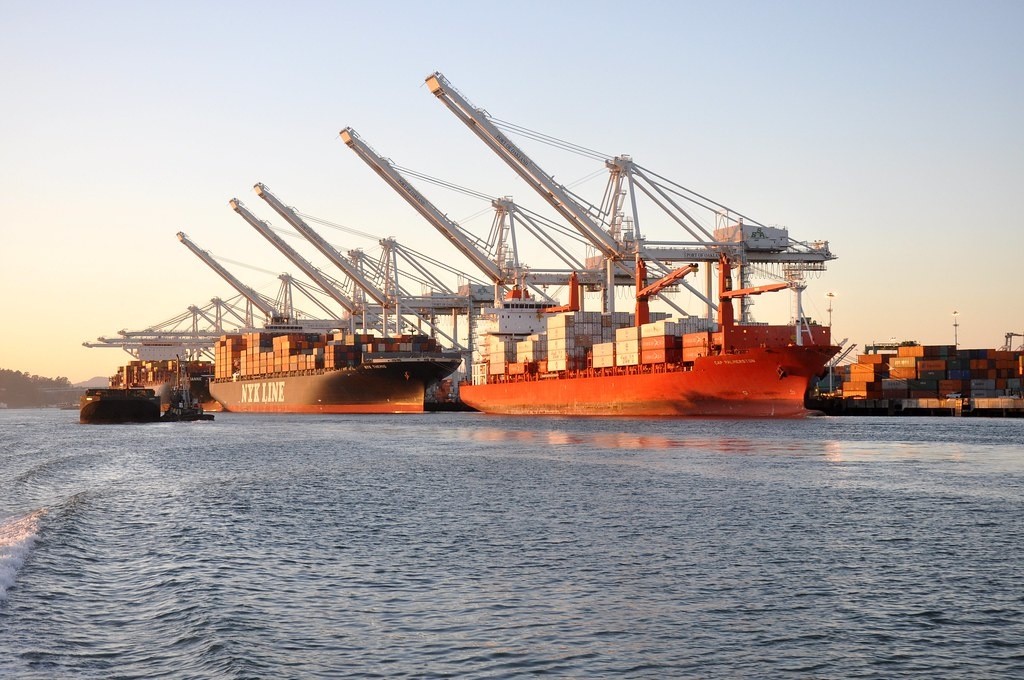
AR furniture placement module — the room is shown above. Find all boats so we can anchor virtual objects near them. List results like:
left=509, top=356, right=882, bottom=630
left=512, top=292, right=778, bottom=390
left=60, top=402, right=79, bottom=410
left=458, top=247, right=845, bottom=419
left=161, top=353, right=215, bottom=422
left=108, top=336, right=224, bottom=412
left=209, top=313, right=463, bottom=415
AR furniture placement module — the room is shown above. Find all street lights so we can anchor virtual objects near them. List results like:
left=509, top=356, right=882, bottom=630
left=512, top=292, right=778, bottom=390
left=827, top=291, right=835, bottom=327
left=953, top=311, right=959, bottom=346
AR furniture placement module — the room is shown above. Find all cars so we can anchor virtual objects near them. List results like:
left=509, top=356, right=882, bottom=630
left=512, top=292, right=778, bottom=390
left=945, top=391, right=962, bottom=398
